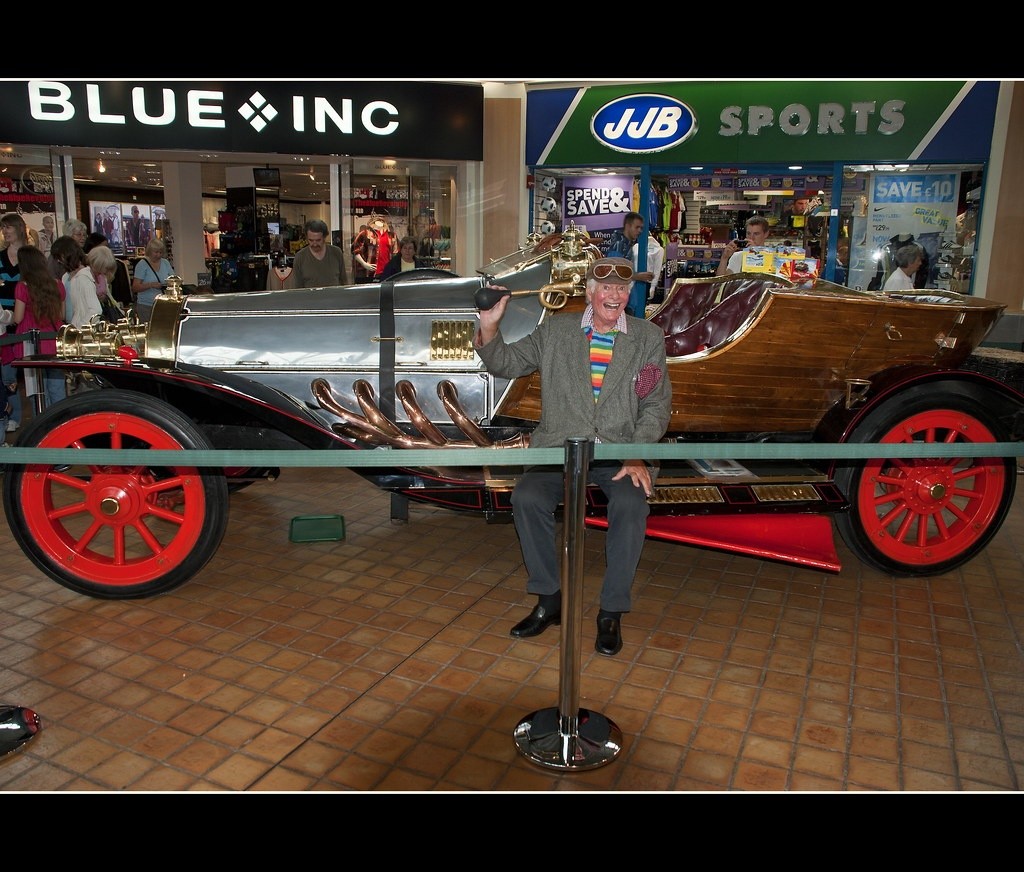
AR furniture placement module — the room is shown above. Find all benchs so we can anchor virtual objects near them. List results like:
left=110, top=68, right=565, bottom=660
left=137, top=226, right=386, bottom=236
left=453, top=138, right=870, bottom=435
left=865, top=288, right=969, bottom=305
left=646, top=271, right=797, bottom=357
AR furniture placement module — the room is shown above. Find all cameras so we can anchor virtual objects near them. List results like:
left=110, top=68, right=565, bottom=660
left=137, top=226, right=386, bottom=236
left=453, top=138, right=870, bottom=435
left=734, top=240, right=747, bottom=248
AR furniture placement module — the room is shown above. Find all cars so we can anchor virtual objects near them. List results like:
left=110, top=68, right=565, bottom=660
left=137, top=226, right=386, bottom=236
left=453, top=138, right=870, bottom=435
left=0, top=217, right=1024, bottom=600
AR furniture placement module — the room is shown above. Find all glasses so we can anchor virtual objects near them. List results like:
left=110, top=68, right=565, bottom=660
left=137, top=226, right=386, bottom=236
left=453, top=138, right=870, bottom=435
left=73, top=232, right=88, bottom=238
left=593, top=265, right=632, bottom=280
left=133, top=210, right=139, bottom=213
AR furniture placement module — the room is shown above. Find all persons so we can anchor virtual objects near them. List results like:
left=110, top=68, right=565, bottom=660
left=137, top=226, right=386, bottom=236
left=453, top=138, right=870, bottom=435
left=780, top=198, right=807, bottom=226
left=717, top=216, right=770, bottom=275
left=605, top=211, right=654, bottom=317
left=632, top=235, right=664, bottom=301
left=0, top=213, right=152, bottom=473
left=38, top=216, right=58, bottom=244
left=882, top=243, right=923, bottom=291
left=471, top=257, right=673, bottom=656
left=291, top=219, right=349, bottom=287
left=132, top=238, right=175, bottom=324
left=352, top=221, right=398, bottom=285
left=820, top=246, right=849, bottom=285
left=94, top=205, right=160, bottom=253
left=866, top=232, right=929, bottom=290
left=381, top=237, right=426, bottom=284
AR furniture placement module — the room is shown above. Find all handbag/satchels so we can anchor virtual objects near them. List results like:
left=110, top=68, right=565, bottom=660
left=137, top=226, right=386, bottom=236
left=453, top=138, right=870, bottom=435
left=99, top=294, right=125, bottom=323
left=161, top=285, right=168, bottom=294
left=155, top=214, right=161, bottom=229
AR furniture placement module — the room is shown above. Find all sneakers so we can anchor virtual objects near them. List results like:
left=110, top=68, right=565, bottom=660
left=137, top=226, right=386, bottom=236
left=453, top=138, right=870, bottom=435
left=5, top=420, right=20, bottom=431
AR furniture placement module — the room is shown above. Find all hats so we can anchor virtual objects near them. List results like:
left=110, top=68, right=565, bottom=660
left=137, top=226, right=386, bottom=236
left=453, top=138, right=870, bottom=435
left=585, top=258, right=634, bottom=285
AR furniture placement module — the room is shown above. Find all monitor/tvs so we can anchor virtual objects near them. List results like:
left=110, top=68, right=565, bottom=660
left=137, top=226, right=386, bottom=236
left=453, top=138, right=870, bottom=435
left=253, top=167, right=281, bottom=187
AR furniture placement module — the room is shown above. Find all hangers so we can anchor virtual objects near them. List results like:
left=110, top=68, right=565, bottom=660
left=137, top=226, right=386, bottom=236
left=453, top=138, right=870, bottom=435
left=277, top=255, right=293, bottom=268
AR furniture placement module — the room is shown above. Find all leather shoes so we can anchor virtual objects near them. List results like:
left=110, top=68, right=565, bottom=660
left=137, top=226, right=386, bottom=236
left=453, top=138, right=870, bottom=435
left=594, top=614, right=623, bottom=656
left=510, top=605, right=561, bottom=637
